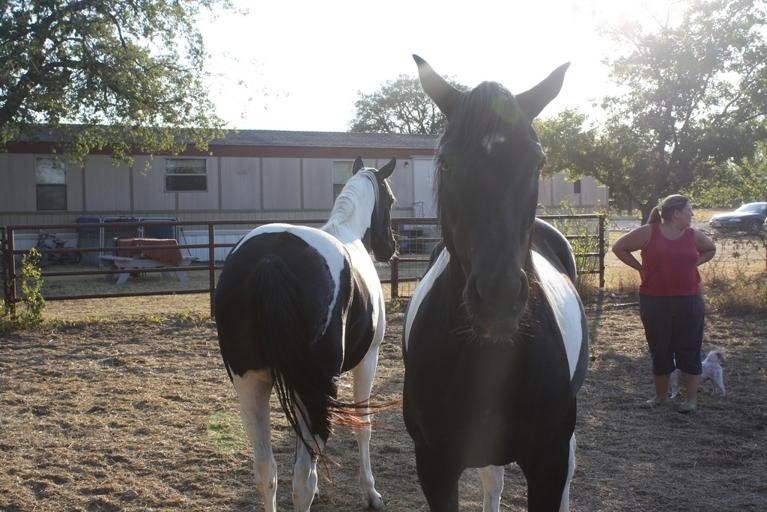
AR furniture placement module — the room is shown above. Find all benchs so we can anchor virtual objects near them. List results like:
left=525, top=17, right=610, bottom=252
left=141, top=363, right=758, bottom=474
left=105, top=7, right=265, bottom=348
left=97, top=254, right=200, bottom=286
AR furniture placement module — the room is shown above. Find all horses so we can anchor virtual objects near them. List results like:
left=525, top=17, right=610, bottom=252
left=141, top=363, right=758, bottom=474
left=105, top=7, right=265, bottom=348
left=402, top=54, right=590, bottom=512
left=214, top=155, right=403, bottom=512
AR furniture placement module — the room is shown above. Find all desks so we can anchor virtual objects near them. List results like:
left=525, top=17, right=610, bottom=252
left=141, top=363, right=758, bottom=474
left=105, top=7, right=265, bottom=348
left=111, top=237, right=188, bottom=285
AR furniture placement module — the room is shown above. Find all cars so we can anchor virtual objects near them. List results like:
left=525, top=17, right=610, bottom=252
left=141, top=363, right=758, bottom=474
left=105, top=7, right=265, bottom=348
left=709, top=201, right=767, bottom=236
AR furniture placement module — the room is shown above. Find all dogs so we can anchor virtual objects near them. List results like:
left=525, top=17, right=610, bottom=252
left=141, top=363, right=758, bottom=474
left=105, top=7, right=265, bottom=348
left=670, top=350, right=726, bottom=399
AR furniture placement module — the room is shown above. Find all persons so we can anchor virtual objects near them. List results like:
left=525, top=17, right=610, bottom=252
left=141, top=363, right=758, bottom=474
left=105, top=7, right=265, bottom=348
left=612, top=194, right=716, bottom=412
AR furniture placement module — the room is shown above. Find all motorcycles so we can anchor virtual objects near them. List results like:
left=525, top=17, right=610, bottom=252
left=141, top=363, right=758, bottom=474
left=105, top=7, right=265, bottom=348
left=29, top=228, right=82, bottom=266
